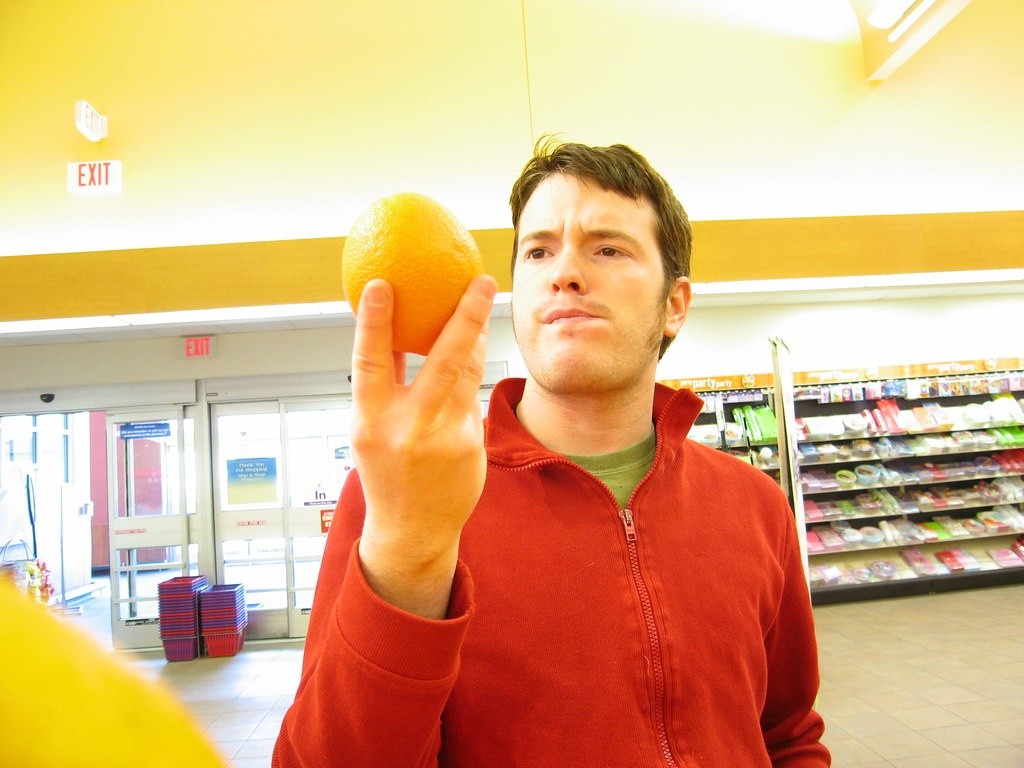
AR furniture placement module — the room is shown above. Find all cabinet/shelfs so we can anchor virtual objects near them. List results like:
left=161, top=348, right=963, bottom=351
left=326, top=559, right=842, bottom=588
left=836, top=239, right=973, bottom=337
left=654, top=335, right=1024, bottom=609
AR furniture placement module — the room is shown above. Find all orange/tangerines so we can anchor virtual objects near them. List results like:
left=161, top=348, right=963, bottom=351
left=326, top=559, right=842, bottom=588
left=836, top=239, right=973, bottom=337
left=341, top=192, right=484, bottom=355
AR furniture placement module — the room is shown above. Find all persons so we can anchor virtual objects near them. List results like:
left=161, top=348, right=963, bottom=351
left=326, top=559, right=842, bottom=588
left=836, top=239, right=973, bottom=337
left=273, top=144, right=837, bottom=768
